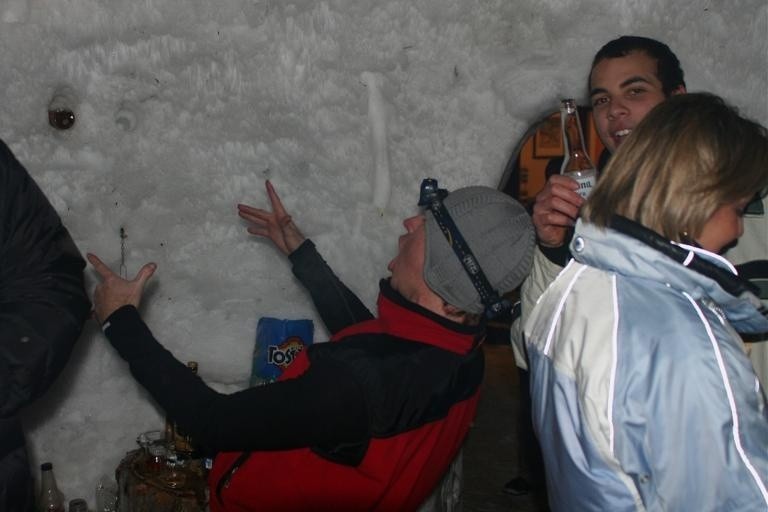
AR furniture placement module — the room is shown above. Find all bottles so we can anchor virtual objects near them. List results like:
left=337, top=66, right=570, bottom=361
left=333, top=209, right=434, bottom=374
left=559, top=98, right=598, bottom=234
left=168, top=358, right=199, bottom=459
left=68, top=498, right=87, bottom=511
left=37, top=463, right=66, bottom=511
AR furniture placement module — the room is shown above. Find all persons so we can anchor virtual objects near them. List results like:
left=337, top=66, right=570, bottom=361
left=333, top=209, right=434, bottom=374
left=510, top=36, right=767, bottom=416
left=84, top=177, right=537, bottom=511
left=0, top=138, right=94, bottom=511
left=519, top=89, right=768, bottom=510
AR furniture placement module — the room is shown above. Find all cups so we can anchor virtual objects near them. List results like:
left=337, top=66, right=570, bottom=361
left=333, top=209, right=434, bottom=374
left=137, top=428, right=194, bottom=489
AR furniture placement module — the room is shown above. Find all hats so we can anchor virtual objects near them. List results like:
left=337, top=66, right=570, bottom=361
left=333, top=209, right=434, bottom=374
left=423, top=186, right=537, bottom=315
